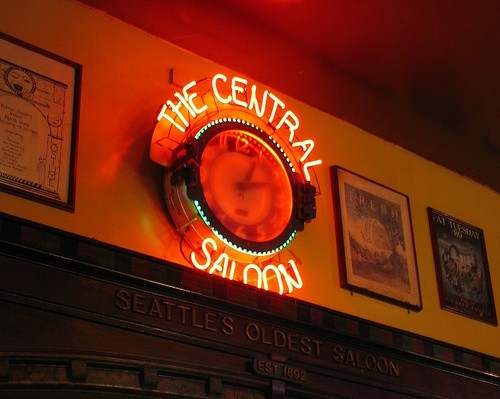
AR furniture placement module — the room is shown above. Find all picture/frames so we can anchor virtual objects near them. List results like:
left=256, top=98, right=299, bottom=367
left=332, top=162, right=424, bottom=311
left=0, top=31, right=84, bottom=212
left=425, top=205, right=498, bottom=325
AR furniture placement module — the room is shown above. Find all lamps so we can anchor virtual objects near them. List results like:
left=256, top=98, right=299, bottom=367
left=149, top=71, right=323, bottom=295
